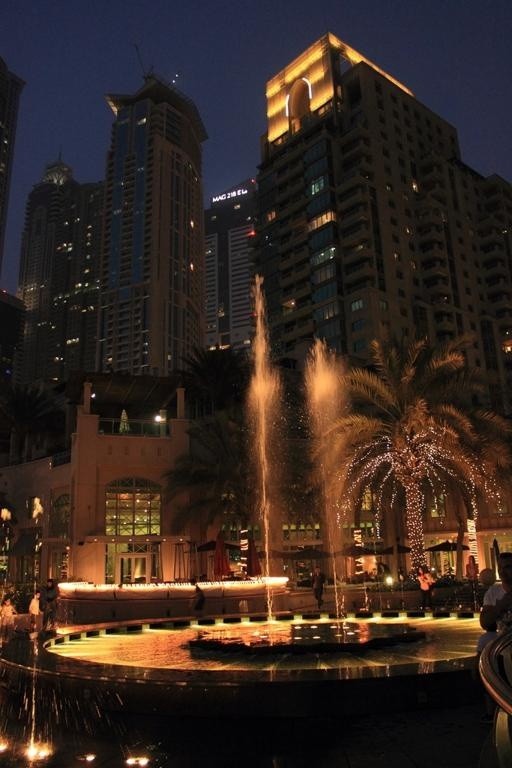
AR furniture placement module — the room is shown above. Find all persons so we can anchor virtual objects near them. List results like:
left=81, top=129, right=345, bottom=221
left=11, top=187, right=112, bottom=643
left=0, top=597, right=16, bottom=642
left=28, top=590, right=43, bottom=632
left=311, top=564, right=327, bottom=608
left=42, top=577, right=59, bottom=630
left=416, top=565, right=435, bottom=607
left=476, top=613, right=503, bottom=723
left=464, top=555, right=480, bottom=599
left=478, top=551, right=512, bottom=633
left=188, top=578, right=206, bottom=618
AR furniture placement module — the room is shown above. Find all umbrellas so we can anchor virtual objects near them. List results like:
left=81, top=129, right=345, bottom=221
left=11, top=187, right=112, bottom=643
left=287, top=547, right=331, bottom=575
left=213, top=530, right=231, bottom=576
left=426, top=541, right=469, bottom=574
left=185, top=540, right=238, bottom=552
left=333, top=543, right=378, bottom=575
left=378, top=543, right=413, bottom=569
left=245, top=528, right=262, bottom=576
left=255, top=549, right=282, bottom=558
